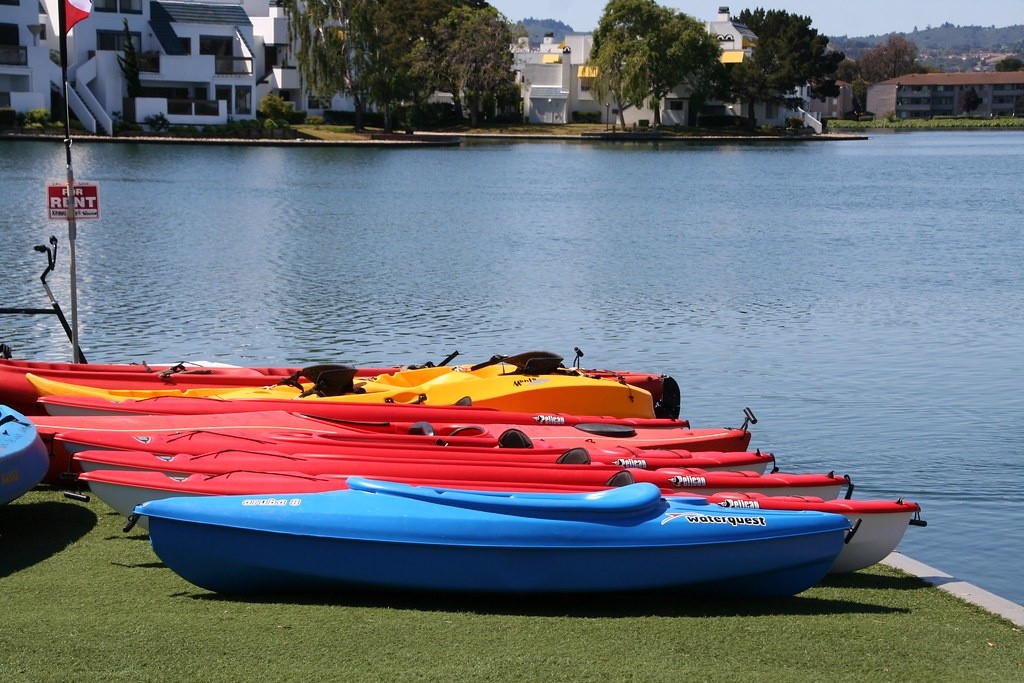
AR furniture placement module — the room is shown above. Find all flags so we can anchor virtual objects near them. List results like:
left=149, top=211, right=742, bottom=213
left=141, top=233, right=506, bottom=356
left=65, top=0, right=92, bottom=36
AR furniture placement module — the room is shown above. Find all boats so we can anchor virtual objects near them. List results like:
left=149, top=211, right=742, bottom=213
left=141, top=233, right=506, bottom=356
left=0, top=344, right=927, bottom=613
